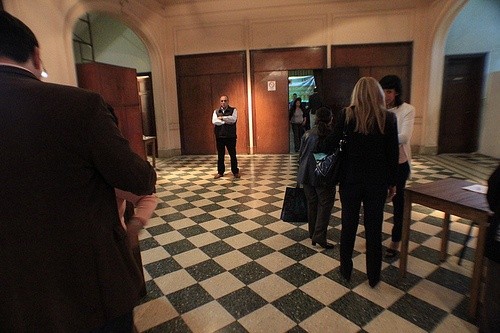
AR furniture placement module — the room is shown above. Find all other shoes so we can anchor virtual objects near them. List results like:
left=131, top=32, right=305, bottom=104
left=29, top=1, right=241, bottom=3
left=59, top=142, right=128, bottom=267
left=214, top=174, right=223, bottom=179
left=341, top=265, right=350, bottom=281
left=368, top=278, right=380, bottom=287
left=235, top=173, right=241, bottom=178
left=385, top=249, right=396, bottom=257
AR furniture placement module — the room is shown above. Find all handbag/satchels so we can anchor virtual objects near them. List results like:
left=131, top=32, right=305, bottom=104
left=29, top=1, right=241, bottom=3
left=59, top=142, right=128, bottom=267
left=280, top=187, right=308, bottom=223
left=314, top=135, right=356, bottom=181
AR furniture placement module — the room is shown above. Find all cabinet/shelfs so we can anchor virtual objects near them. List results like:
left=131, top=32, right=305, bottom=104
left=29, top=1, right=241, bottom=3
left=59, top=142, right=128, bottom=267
left=76, top=61, right=144, bottom=162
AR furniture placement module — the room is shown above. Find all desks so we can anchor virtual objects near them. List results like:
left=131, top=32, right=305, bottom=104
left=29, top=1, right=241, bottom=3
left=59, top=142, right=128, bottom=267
left=144, top=136, right=156, bottom=168
left=398, top=177, right=494, bottom=318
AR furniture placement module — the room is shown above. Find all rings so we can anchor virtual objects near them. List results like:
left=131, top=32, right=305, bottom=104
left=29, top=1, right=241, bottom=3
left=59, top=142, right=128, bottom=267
left=392, top=192, right=396, bottom=195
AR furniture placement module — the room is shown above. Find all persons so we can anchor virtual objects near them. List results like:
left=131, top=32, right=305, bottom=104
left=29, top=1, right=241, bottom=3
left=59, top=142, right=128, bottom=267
left=0, top=9, right=158, bottom=333
left=289, top=89, right=349, bottom=152
left=297, top=107, right=339, bottom=248
left=211, top=95, right=241, bottom=178
left=483, top=165, right=500, bottom=333
left=379, top=75, right=416, bottom=257
left=335, top=76, right=399, bottom=289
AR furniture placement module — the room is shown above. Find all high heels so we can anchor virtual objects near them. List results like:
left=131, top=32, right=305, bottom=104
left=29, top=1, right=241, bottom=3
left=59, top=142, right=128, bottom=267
left=309, top=235, right=312, bottom=239
left=312, top=239, right=334, bottom=249
